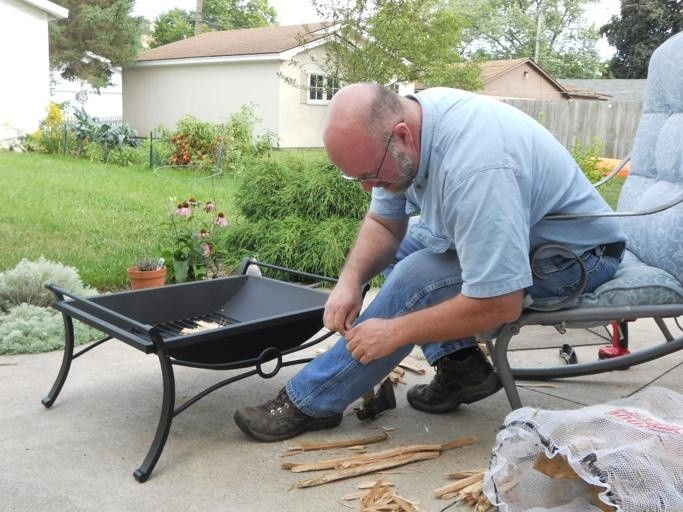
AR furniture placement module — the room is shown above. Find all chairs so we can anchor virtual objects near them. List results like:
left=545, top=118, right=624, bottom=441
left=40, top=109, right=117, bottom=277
left=478, top=28, right=683, bottom=421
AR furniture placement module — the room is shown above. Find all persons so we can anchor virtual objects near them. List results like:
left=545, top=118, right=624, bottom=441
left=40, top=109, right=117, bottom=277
left=234, top=82, right=627, bottom=442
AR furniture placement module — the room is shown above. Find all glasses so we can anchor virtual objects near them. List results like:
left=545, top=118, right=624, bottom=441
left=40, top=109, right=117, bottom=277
left=339, top=119, right=405, bottom=183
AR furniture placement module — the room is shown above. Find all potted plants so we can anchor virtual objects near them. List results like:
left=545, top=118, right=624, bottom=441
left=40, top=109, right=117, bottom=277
left=124, top=255, right=167, bottom=290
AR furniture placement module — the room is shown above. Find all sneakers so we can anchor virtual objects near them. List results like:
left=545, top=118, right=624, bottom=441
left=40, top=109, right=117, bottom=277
left=406, top=348, right=505, bottom=413
left=233, top=379, right=344, bottom=442
